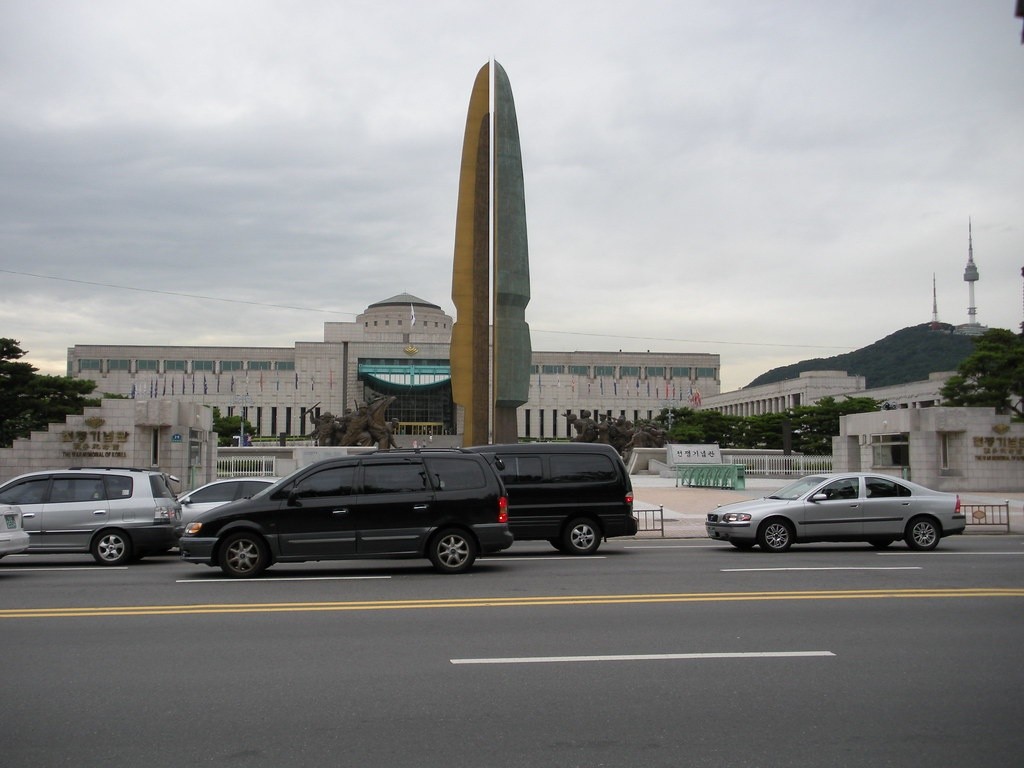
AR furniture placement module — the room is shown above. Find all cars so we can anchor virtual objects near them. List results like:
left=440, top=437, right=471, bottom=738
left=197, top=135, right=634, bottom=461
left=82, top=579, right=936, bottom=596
left=705, top=472, right=965, bottom=553
left=0, top=503, right=31, bottom=561
left=174, top=476, right=285, bottom=549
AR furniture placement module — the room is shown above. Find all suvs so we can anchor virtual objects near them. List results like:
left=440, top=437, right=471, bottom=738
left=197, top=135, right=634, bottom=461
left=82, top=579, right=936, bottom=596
left=177, top=448, right=514, bottom=578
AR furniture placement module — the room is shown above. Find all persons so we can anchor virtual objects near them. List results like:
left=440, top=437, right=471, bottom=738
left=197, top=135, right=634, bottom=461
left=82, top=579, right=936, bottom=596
left=561, top=410, right=669, bottom=466
left=305, top=395, right=403, bottom=454
left=412, top=435, right=432, bottom=449
left=824, top=481, right=845, bottom=501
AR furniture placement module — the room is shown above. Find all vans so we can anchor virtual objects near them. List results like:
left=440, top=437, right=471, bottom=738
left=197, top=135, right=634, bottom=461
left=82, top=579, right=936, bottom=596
left=0, top=467, right=179, bottom=568
left=458, top=442, right=638, bottom=556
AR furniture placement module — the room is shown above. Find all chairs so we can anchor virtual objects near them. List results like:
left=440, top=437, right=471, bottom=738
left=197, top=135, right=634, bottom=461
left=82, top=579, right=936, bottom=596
left=844, top=480, right=856, bottom=497
